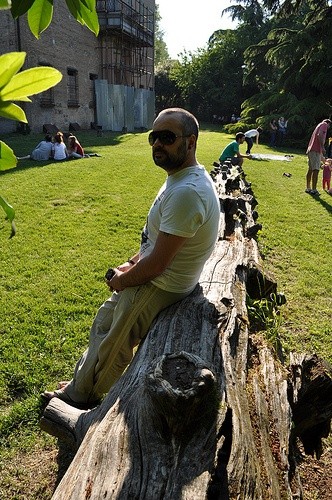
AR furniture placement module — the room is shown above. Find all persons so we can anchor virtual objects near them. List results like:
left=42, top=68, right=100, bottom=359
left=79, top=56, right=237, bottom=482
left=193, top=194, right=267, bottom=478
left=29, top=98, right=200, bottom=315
left=212, top=114, right=332, bottom=196
left=16, top=131, right=83, bottom=161
left=41, top=108, right=219, bottom=417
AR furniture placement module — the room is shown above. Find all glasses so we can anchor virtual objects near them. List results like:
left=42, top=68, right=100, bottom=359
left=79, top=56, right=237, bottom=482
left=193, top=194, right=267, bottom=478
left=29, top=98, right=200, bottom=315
left=148, top=130, right=194, bottom=147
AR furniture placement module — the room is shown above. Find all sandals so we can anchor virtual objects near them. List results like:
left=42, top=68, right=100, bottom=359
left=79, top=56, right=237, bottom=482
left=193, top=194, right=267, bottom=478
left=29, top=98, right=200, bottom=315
left=40, top=390, right=80, bottom=409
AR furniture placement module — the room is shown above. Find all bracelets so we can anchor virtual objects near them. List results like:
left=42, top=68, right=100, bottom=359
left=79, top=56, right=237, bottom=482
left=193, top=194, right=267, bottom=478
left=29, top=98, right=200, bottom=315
left=126, top=260, right=135, bottom=265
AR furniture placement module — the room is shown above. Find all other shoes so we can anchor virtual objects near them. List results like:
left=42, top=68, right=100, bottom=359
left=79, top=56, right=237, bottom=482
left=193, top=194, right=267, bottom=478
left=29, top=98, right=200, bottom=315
left=305, top=189, right=311, bottom=193
left=310, top=191, right=319, bottom=195
